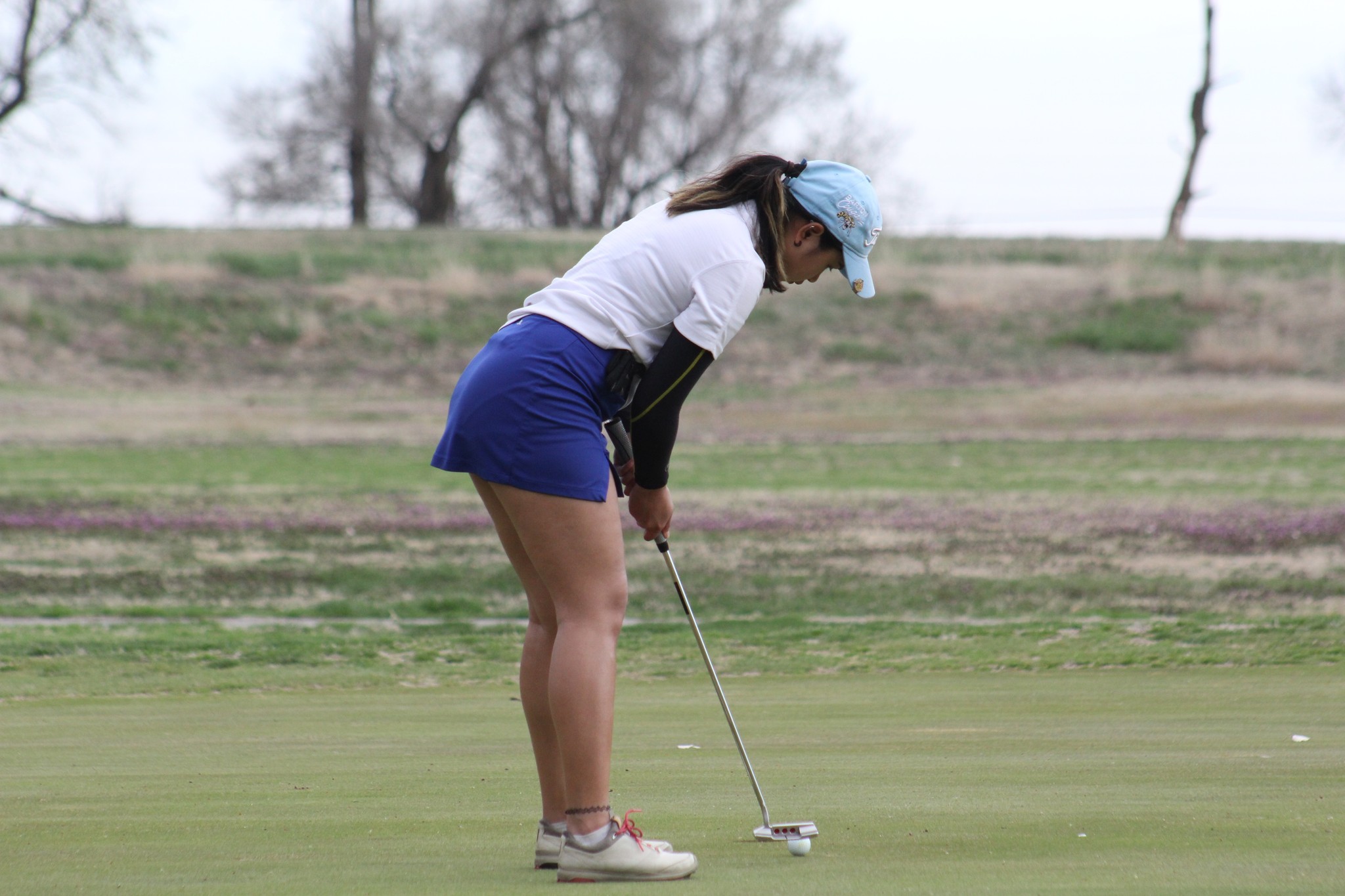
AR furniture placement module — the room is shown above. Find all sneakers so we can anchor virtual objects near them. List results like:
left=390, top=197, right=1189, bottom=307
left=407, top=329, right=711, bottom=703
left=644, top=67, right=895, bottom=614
left=557, top=809, right=699, bottom=882
left=534, top=820, right=673, bottom=869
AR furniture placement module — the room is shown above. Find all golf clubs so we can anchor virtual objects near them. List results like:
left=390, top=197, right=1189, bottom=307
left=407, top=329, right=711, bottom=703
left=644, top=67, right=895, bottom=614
left=606, top=415, right=820, bottom=840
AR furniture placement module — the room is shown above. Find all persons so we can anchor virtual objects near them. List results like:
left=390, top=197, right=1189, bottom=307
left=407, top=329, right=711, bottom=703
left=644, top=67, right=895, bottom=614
left=430, top=156, right=881, bottom=881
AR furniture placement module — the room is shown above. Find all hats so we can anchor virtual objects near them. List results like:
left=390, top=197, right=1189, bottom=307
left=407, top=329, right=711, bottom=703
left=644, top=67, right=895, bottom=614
left=782, top=160, right=882, bottom=299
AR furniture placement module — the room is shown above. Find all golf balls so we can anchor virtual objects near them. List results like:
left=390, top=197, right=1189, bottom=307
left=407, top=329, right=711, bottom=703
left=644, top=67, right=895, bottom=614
left=786, top=833, right=812, bottom=857
